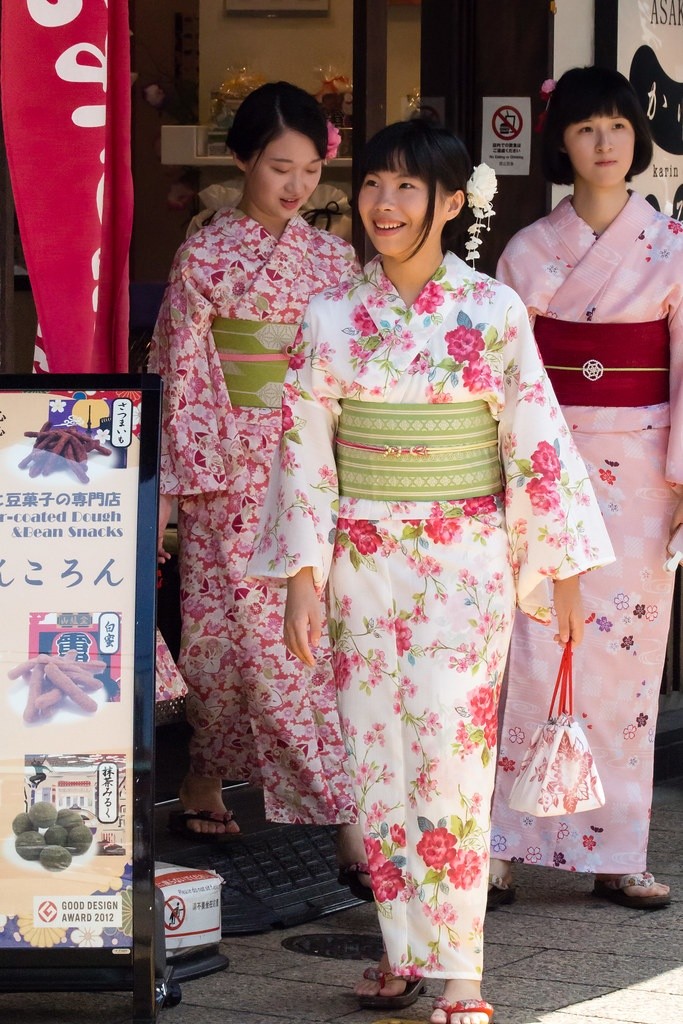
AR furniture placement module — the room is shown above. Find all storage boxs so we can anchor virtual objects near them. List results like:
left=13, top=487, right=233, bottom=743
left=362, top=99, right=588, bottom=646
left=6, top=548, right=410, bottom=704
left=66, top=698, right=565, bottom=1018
left=154, top=861, right=224, bottom=958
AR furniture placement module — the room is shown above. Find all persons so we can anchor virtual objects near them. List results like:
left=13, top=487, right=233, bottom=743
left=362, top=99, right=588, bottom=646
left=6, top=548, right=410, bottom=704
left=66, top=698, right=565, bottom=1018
left=284, top=119, right=590, bottom=1023
left=143, top=81, right=375, bottom=902
left=496, top=64, right=683, bottom=911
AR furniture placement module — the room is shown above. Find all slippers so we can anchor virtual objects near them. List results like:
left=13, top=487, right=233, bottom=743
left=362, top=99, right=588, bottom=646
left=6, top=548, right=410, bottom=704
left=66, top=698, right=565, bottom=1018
left=166, top=809, right=243, bottom=843
left=593, top=872, right=670, bottom=909
left=359, top=966, right=427, bottom=1012
left=337, top=862, right=376, bottom=904
left=433, top=994, right=494, bottom=1024
left=486, top=873, right=515, bottom=911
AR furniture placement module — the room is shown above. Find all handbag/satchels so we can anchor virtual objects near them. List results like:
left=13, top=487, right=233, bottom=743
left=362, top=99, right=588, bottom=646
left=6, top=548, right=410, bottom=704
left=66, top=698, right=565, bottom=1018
left=155, top=624, right=188, bottom=702
left=508, top=640, right=607, bottom=817
